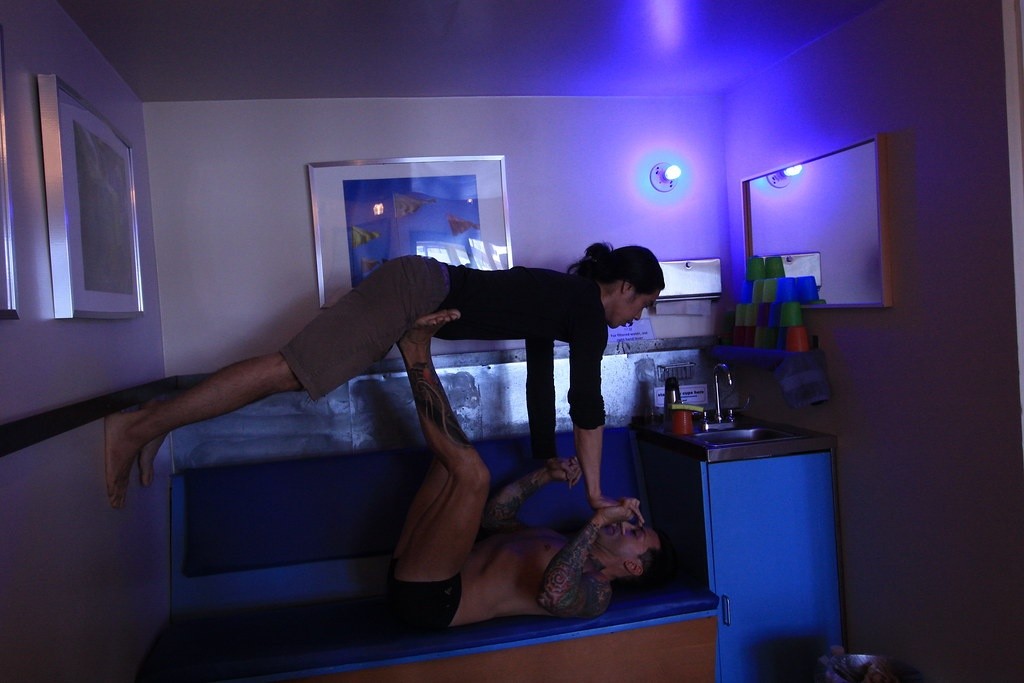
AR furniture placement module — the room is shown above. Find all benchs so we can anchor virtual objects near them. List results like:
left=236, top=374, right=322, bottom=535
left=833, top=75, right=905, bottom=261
left=138, top=429, right=721, bottom=683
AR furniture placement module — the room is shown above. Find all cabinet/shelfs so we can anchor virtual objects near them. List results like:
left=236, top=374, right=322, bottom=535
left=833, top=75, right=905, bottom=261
left=628, top=437, right=849, bottom=683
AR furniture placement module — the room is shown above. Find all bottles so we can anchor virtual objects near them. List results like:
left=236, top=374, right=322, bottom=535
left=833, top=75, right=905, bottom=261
left=662, top=376, right=685, bottom=431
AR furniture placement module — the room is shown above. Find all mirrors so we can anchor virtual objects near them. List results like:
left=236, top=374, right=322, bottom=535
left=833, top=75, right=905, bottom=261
left=740, top=131, right=893, bottom=309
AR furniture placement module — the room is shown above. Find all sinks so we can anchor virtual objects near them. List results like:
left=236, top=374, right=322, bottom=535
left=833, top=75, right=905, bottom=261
left=696, top=427, right=802, bottom=449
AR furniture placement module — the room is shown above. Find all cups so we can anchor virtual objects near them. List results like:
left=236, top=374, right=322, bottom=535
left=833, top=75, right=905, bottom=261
left=796, top=274, right=826, bottom=304
left=765, top=257, right=785, bottom=278
left=672, top=409, right=693, bottom=435
left=729, top=256, right=809, bottom=353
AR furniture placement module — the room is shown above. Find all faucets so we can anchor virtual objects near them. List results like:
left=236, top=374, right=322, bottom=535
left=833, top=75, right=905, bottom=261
left=713, top=361, right=733, bottom=421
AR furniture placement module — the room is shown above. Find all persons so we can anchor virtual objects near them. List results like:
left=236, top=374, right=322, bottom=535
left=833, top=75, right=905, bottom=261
left=380, top=307, right=664, bottom=635
left=103, top=241, right=665, bottom=526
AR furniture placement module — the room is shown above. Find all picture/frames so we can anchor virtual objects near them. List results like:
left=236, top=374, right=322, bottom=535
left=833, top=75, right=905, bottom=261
left=36, top=72, right=147, bottom=319
left=306, top=155, right=514, bottom=310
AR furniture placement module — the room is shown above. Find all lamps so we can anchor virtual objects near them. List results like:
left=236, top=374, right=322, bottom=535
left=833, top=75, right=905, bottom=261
left=649, top=163, right=682, bottom=193
left=766, top=164, right=803, bottom=189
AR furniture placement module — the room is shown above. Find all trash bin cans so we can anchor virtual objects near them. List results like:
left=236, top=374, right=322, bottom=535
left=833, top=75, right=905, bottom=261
left=818, top=645, right=925, bottom=683
